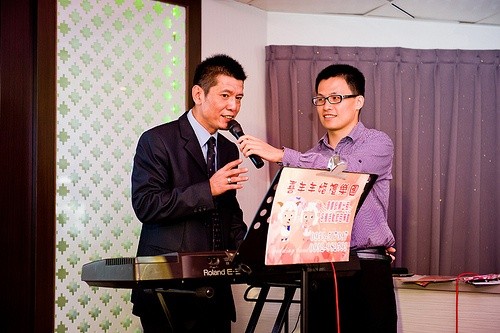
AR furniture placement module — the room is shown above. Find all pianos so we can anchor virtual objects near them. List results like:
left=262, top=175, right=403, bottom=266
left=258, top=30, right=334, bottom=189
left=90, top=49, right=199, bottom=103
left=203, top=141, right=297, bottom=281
left=80, top=250, right=362, bottom=333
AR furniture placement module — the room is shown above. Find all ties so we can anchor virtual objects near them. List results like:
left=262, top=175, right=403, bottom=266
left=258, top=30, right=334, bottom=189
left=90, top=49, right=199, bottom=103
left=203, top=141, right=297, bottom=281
left=202, top=138, right=223, bottom=252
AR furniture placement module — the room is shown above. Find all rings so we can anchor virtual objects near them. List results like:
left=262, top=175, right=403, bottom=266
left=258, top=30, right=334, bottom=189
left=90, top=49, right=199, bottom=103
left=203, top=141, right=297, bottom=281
left=227, top=176, right=230, bottom=184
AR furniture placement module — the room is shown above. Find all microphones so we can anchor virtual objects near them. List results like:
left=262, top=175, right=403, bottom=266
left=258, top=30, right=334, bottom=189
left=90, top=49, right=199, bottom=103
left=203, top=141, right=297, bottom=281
left=227, top=120, right=265, bottom=169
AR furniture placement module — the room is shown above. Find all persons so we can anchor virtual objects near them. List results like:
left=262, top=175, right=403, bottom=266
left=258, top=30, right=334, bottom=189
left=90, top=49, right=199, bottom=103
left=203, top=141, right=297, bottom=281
left=236, top=64, right=398, bottom=333
left=131, top=54, right=249, bottom=333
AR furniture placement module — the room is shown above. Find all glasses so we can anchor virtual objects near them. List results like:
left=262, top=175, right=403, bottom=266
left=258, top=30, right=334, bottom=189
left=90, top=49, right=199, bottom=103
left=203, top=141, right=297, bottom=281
left=312, top=94, right=359, bottom=107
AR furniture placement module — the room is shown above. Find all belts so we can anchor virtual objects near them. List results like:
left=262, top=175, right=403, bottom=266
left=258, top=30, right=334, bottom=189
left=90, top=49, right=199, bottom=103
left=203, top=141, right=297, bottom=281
left=358, top=246, right=393, bottom=262
